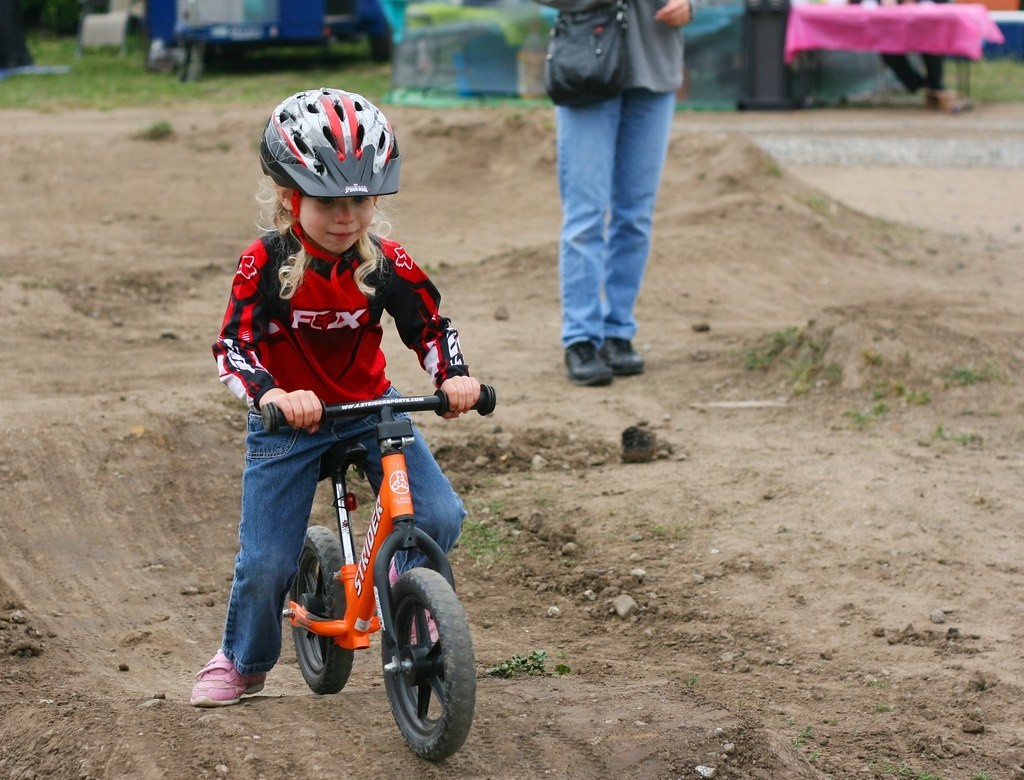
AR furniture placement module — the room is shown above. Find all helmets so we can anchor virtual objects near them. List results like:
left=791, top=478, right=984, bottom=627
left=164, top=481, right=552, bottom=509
left=259, top=87, right=403, bottom=197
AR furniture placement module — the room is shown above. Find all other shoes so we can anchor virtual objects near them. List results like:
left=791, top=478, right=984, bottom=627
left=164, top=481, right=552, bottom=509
left=564, top=341, right=613, bottom=387
left=597, top=337, right=645, bottom=374
left=925, top=89, right=970, bottom=111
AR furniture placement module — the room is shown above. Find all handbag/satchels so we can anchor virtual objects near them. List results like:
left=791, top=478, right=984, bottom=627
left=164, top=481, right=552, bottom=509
left=544, top=0, right=628, bottom=107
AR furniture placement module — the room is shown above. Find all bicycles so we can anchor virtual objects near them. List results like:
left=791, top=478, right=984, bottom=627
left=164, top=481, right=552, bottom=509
left=260, top=384, right=497, bottom=763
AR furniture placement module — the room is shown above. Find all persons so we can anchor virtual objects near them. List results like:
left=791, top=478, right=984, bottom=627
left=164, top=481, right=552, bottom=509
left=532, top=1, right=694, bottom=386
left=189, top=88, right=481, bottom=707
left=879, top=0, right=956, bottom=100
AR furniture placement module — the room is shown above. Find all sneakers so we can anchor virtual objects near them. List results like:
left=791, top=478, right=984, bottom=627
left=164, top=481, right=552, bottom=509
left=387, top=553, right=439, bottom=646
left=190, top=648, right=267, bottom=707
